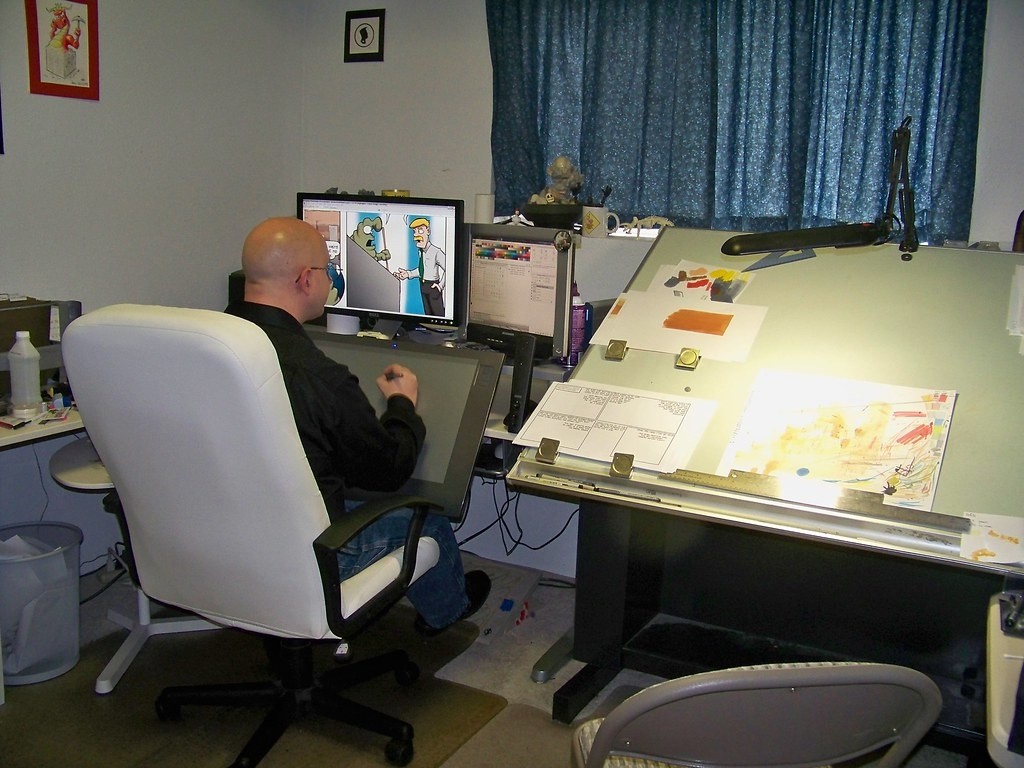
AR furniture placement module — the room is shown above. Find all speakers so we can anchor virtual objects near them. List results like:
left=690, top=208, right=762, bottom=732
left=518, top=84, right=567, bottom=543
left=508, top=334, right=536, bottom=433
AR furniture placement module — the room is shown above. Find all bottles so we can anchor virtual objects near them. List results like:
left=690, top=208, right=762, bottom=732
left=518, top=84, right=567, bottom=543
left=8, top=332, right=43, bottom=404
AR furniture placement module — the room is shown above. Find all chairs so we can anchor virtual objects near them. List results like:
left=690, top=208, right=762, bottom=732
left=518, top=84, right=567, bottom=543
left=572, top=661, right=943, bottom=768
left=61, top=303, right=444, bottom=768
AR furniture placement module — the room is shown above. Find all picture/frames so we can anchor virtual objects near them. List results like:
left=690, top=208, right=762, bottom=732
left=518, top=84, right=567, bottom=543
left=344, top=8, right=385, bottom=63
left=25, top=0, right=99, bottom=100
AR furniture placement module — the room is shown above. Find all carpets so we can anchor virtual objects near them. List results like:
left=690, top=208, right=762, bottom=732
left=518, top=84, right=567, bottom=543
left=0, top=610, right=508, bottom=768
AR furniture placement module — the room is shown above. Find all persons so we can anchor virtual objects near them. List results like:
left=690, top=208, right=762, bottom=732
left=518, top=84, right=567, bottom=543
left=222, top=218, right=491, bottom=636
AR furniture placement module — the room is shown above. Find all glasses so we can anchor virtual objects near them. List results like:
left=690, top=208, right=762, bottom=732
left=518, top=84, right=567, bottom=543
left=295, top=262, right=344, bottom=283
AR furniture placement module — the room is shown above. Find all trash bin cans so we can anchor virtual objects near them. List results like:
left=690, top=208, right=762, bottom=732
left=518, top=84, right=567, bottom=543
left=0, top=521, right=84, bottom=683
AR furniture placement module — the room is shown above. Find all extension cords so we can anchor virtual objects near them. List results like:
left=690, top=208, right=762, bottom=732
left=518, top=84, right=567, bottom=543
left=98, top=568, right=130, bottom=584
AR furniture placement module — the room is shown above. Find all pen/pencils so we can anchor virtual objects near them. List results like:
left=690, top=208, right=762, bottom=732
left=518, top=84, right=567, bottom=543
left=10, top=410, right=50, bottom=431
left=525, top=472, right=662, bottom=504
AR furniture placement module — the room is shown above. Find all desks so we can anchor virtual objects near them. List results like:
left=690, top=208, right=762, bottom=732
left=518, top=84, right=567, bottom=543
left=407, top=330, right=573, bottom=684
left=0, top=401, right=86, bottom=452
left=49, top=438, right=232, bottom=694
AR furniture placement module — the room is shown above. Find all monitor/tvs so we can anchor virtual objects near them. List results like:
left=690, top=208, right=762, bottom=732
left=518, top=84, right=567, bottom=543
left=464, top=223, right=576, bottom=363
left=295, top=191, right=465, bottom=340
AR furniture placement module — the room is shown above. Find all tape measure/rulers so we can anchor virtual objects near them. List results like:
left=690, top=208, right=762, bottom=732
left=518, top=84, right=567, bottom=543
left=741, top=249, right=816, bottom=272
left=657, top=467, right=971, bottom=532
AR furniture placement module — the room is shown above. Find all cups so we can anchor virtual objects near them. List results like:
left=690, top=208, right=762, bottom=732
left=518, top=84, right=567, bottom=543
left=561, top=281, right=593, bottom=367
left=582, top=206, right=619, bottom=236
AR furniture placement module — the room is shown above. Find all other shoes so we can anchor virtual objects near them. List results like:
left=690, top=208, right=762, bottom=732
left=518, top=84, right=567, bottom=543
left=413, top=569, right=493, bottom=637
